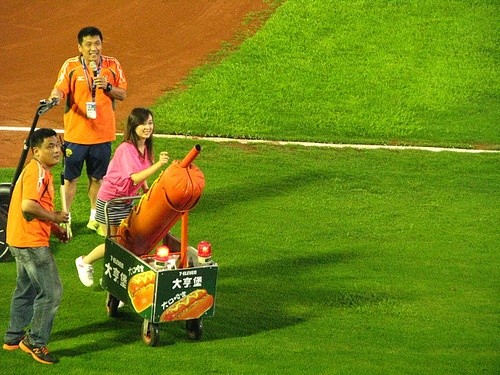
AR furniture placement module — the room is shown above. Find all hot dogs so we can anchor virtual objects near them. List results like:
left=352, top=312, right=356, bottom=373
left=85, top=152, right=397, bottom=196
left=160, top=289, right=213, bottom=322
left=128, top=270, right=155, bottom=313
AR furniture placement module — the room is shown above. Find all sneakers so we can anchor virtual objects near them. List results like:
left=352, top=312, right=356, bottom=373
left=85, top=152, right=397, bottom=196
left=87, top=219, right=105, bottom=236
left=19, top=336, right=56, bottom=364
left=3, top=336, right=24, bottom=350
left=75, top=255, right=93, bottom=287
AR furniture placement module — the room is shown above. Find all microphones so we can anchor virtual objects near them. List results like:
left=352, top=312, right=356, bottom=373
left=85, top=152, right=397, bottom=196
left=90, top=61, right=102, bottom=89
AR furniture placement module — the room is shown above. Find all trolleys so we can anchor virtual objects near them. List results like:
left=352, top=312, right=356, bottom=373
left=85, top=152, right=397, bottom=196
left=99, top=194, right=220, bottom=347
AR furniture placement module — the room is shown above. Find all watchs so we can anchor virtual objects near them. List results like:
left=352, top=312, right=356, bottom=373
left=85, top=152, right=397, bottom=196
left=103, top=82, right=112, bottom=92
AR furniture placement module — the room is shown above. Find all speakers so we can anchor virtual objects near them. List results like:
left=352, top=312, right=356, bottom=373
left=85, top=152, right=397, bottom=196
left=0, top=182, right=15, bottom=263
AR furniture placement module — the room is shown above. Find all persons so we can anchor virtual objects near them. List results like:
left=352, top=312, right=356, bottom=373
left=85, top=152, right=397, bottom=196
left=75, top=107, right=169, bottom=309
left=46, top=26, right=127, bottom=238
left=3, top=128, right=73, bottom=365
